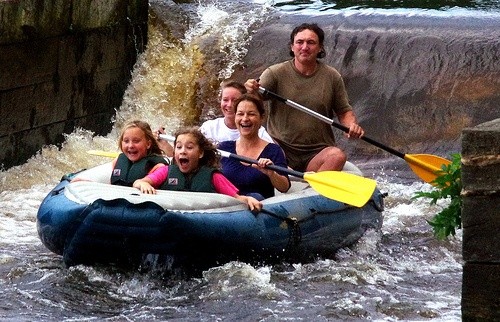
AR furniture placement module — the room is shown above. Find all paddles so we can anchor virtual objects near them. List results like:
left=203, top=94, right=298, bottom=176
left=87, top=149, right=120, bottom=159
left=258, top=79, right=459, bottom=188
left=156, top=129, right=377, bottom=208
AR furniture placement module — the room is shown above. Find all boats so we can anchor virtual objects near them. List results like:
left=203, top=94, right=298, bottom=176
left=36, top=151, right=387, bottom=278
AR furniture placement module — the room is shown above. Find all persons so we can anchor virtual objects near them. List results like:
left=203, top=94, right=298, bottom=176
left=198, top=81, right=275, bottom=146
left=244, top=23, right=364, bottom=173
left=133, top=129, right=263, bottom=213
left=152, top=93, right=291, bottom=201
left=69, top=120, right=169, bottom=187
